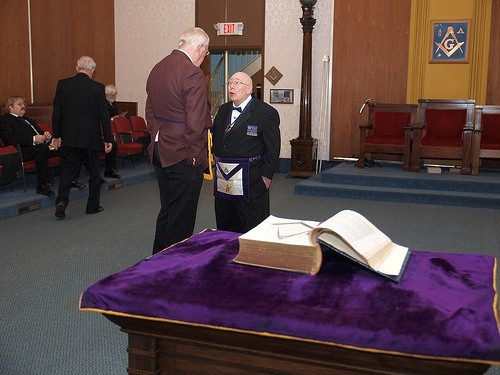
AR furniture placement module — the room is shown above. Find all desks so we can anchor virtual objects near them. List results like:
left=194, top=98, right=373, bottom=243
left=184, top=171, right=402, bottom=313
left=79, top=228, right=500, bottom=375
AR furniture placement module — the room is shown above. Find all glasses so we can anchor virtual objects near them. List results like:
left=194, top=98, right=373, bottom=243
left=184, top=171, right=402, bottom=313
left=17, top=103, right=27, bottom=107
left=228, top=80, right=249, bottom=86
left=198, top=43, right=210, bottom=56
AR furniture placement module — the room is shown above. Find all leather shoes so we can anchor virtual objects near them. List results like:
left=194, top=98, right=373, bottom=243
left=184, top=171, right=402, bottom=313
left=72, top=180, right=86, bottom=188
left=36, top=183, right=55, bottom=196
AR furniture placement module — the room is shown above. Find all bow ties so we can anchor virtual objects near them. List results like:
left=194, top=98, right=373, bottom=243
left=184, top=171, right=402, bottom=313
left=231, top=106, right=241, bottom=112
left=18, top=116, right=24, bottom=119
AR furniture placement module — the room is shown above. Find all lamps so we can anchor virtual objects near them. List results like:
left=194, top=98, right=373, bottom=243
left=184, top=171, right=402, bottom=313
left=213, top=22, right=244, bottom=36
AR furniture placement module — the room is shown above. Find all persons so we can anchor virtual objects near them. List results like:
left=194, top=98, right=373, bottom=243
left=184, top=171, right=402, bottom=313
left=145, top=27, right=213, bottom=255
left=210, top=72, right=281, bottom=234
left=0, top=96, right=86, bottom=195
left=51, top=56, right=114, bottom=218
left=101, top=84, right=120, bottom=178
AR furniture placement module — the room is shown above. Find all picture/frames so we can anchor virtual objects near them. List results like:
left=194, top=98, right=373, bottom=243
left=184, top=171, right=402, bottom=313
left=270, top=89, right=294, bottom=104
left=429, top=19, right=472, bottom=64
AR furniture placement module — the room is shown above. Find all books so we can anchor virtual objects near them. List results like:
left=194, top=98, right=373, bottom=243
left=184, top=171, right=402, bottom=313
left=229, top=209, right=413, bottom=284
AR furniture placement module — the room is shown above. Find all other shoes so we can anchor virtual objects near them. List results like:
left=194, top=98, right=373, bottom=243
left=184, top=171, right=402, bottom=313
left=98, top=206, right=104, bottom=212
left=100, top=179, right=107, bottom=184
left=55, top=201, right=66, bottom=217
left=105, top=173, right=120, bottom=178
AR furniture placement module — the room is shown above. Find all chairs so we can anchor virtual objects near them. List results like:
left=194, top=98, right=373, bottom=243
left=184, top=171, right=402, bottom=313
left=470, top=105, right=500, bottom=176
left=0, top=116, right=148, bottom=192
left=358, top=103, right=418, bottom=171
left=411, top=99, right=476, bottom=175
